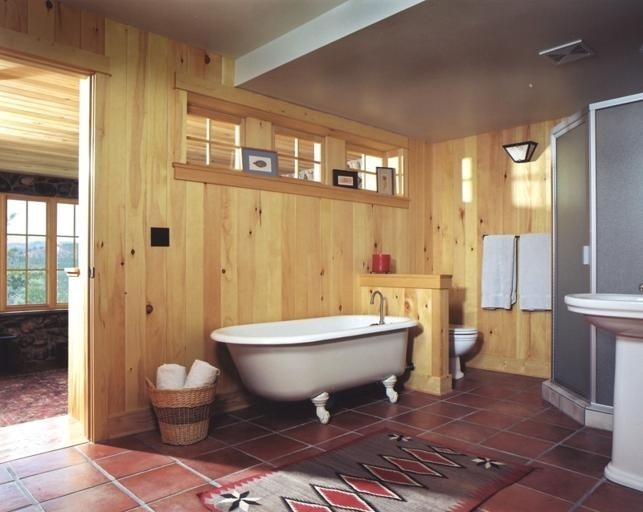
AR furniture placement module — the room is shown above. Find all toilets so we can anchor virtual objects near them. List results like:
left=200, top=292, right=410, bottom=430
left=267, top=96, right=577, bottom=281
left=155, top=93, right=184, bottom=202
left=448, top=328, right=479, bottom=380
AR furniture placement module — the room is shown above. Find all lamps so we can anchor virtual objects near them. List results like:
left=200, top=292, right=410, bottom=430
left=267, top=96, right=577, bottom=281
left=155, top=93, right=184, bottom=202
left=502, top=124, right=539, bottom=163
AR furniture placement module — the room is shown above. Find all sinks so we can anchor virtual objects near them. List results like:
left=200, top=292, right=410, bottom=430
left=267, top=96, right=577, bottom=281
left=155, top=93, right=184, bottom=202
left=564, top=293, right=643, bottom=338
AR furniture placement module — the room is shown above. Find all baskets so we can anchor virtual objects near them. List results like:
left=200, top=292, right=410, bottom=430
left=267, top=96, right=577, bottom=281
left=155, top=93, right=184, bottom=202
left=142, top=374, right=219, bottom=447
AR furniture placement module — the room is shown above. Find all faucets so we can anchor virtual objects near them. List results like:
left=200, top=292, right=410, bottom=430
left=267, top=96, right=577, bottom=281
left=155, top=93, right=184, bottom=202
left=370, top=291, right=383, bottom=324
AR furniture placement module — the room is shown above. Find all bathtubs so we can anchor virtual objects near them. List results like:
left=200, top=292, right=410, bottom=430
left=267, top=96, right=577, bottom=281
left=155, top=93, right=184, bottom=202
left=211, top=314, right=419, bottom=424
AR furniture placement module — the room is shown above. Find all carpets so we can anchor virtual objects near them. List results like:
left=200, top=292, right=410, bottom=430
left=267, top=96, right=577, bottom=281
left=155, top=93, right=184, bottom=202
left=194, top=427, right=537, bottom=512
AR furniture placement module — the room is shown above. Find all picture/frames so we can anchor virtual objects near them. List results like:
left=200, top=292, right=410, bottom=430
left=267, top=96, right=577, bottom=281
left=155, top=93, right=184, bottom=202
left=242, top=147, right=278, bottom=176
left=333, top=168, right=358, bottom=189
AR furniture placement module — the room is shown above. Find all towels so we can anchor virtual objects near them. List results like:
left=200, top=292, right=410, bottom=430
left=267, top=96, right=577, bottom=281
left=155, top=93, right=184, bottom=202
left=517, top=231, right=553, bottom=311
left=480, top=234, right=517, bottom=310
